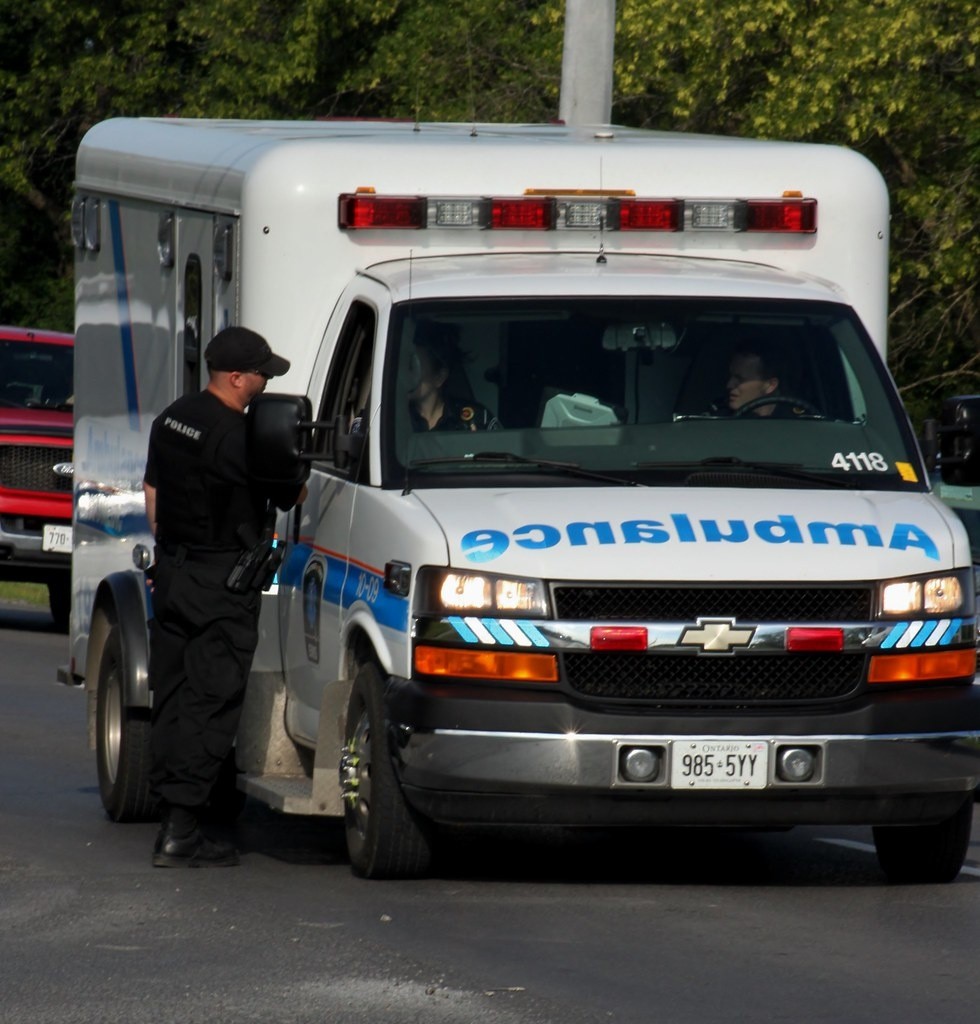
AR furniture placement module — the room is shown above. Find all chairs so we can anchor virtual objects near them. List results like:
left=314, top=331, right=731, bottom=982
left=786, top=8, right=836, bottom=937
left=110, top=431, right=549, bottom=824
left=462, top=379, right=499, bottom=417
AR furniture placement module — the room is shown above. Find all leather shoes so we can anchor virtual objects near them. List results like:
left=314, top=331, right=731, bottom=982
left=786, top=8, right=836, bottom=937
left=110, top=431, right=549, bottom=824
left=150, top=828, right=239, bottom=867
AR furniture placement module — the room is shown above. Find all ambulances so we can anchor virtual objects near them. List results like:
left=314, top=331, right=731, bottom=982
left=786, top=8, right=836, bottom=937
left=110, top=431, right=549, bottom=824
left=53, top=117, right=980, bottom=888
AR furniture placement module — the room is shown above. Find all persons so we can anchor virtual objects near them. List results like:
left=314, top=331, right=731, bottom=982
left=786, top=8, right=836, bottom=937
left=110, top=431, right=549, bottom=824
left=143, top=327, right=308, bottom=868
left=28, top=348, right=74, bottom=405
left=702, top=343, right=825, bottom=420
left=351, top=337, right=505, bottom=459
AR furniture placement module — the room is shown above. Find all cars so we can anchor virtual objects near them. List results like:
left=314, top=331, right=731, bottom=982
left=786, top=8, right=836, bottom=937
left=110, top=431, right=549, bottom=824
left=0, top=326, right=75, bottom=625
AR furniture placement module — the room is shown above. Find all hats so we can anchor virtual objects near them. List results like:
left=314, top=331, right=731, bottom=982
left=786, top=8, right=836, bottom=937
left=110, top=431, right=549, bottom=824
left=204, top=326, right=290, bottom=377
